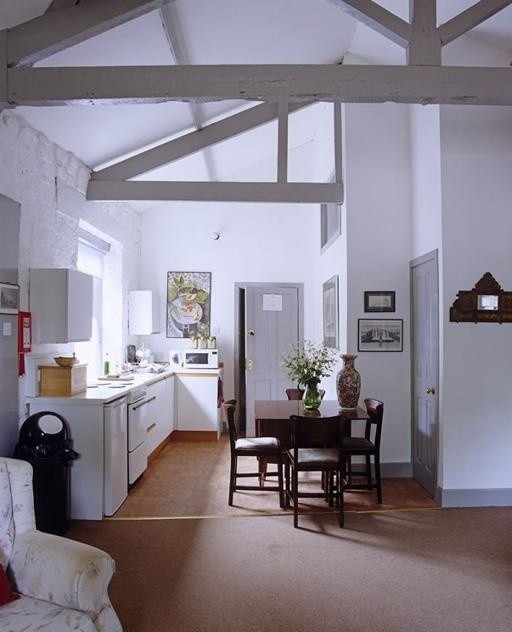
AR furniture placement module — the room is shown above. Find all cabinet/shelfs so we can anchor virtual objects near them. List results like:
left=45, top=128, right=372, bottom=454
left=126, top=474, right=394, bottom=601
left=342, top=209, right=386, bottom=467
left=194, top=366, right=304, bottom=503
left=30, top=267, right=93, bottom=344
left=24, top=396, right=128, bottom=517
left=148, top=375, right=174, bottom=458
left=174, top=374, right=220, bottom=442
left=129, top=290, right=161, bottom=336
left=128, top=385, right=147, bottom=486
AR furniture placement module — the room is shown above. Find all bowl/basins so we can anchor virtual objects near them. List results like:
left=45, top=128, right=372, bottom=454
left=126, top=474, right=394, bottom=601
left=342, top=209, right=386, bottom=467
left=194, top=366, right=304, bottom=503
left=54, top=357, right=77, bottom=367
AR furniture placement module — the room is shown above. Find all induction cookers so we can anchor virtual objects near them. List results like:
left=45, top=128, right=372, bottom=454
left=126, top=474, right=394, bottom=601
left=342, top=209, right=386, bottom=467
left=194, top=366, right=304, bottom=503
left=87, top=381, right=148, bottom=404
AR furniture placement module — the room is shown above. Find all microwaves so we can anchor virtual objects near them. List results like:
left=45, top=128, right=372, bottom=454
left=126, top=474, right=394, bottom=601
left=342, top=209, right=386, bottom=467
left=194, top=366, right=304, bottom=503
left=184, top=349, right=219, bottom=369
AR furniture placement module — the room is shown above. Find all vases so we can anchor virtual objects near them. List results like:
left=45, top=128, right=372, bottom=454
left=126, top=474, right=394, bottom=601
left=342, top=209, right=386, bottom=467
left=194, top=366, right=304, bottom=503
left=336, top=354, right=362, bottom=410
left=303, top=381, right=321, bottom=410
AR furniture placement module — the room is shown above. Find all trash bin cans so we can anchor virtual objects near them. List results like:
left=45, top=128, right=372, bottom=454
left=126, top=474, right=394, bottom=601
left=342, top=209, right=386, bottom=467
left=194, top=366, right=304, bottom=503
left=14, top=411, right=81, bottom=536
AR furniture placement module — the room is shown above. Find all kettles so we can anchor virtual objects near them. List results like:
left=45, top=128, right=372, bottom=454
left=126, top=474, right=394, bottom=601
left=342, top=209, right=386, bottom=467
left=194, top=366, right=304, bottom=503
left=169, top=351, right=183, bottom=368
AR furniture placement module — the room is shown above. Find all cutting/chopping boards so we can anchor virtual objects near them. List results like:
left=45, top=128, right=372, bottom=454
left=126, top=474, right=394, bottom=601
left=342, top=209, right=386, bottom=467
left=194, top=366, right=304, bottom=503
left=97, top=376, right=134, bottom=381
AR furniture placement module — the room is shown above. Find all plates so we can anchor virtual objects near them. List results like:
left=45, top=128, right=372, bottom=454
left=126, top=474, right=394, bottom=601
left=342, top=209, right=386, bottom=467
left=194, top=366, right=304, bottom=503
left=170, top=298, right=202, bottom=325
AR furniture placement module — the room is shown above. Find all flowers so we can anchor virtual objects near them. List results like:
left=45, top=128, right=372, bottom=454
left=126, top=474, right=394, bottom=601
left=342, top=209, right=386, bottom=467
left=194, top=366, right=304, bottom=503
left=282, top=339, right=336, bottom=385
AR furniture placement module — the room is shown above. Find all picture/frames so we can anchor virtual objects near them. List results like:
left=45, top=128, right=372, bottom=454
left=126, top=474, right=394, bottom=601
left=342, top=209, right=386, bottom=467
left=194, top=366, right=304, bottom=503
left=322, top=272, right=339, bottom=353
left=166, top=271, right=211, bottom=339
left=0, top=282, right=20, bottom=316
left=357, top=318, right=404, bottom=352
left=364, top=290, right=396, bottom=313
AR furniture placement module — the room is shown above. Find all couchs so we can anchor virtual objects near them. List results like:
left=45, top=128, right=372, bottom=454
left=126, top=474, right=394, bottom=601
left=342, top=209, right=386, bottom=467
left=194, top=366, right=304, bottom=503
left=2, top=456, right=125, bottom=630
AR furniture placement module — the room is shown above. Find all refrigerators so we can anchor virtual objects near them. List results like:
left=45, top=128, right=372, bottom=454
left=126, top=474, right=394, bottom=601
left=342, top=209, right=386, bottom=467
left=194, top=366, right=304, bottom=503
left=126, top=397, right=148, bottom=487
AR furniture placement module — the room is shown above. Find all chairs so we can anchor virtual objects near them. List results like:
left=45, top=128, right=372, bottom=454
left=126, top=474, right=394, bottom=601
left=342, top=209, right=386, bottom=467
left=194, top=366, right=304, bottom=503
left=222, top=399, right=285, bottom=509
left=322, top=399, right=384, bottom=504
left=284, top=414, right=347, bottom=528
left=286, top=388, right=325, bottom=401
